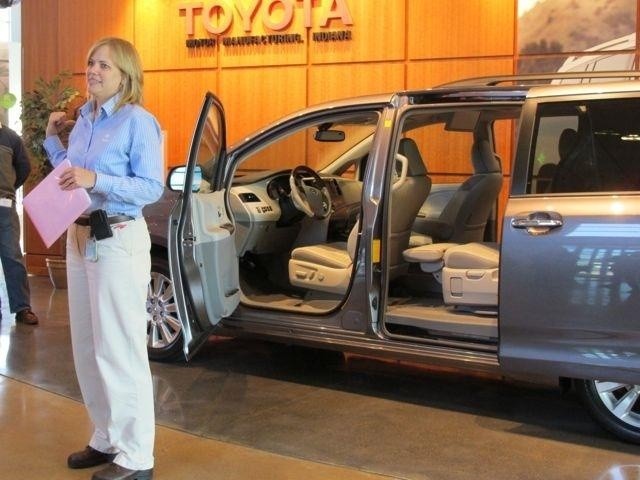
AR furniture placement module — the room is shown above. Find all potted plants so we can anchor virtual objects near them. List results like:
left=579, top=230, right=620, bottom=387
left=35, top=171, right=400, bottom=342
left=15, top=73, right=85, bottom=289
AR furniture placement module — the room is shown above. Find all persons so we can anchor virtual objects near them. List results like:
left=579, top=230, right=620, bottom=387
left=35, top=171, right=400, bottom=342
left=0, top=119, right=39, bottom=326
left=42, top=38, right=165, bottom=479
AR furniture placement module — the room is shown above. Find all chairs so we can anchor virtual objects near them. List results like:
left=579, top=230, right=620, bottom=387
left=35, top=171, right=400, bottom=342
left=536, top=128, right=578, bottom=193
left=402, top=244, right=500, bottom=307
left=412, top=140, right=503, bottom=243
left=288, top=138, right=433, bottom=295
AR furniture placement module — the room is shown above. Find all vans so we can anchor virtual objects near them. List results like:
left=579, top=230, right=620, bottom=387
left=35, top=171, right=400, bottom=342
left=142, top=70, right=640, bottom=445
left=551, top=31, right=638, bottom=85
left=146, top=359, right=640, bottom=480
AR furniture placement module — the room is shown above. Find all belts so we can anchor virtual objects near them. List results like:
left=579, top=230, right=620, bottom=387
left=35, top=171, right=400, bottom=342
left=74, top=214, right=134, bottom=227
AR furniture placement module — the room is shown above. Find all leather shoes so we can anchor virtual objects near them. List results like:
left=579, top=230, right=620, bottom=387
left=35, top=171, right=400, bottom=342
left=68, top=445, right=117, bottom=468
left=18, top=308, right=37, bottom=323
left=92, top=463, right=152, bottom=480
